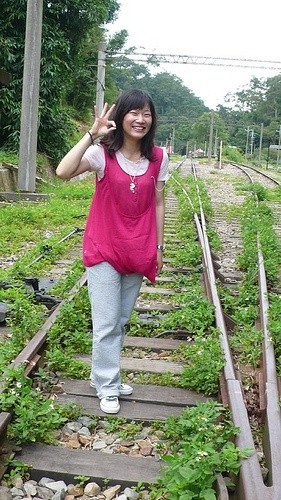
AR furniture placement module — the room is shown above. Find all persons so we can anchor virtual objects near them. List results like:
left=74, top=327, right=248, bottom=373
left=56, top=89, right=169, bottom=413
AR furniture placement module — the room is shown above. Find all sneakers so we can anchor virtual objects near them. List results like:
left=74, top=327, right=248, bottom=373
left=100, top=394, right=120, bottom=414
left=90, top=372, right=133, bottom=395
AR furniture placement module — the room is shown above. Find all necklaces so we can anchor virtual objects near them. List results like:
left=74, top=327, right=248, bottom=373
left=121, top=146, right=143, bottom=193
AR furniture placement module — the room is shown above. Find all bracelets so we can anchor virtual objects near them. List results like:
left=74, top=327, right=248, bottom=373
left=87, top=131, right=94, bottom=144
left=157, top=244, right=165, bottom=250
